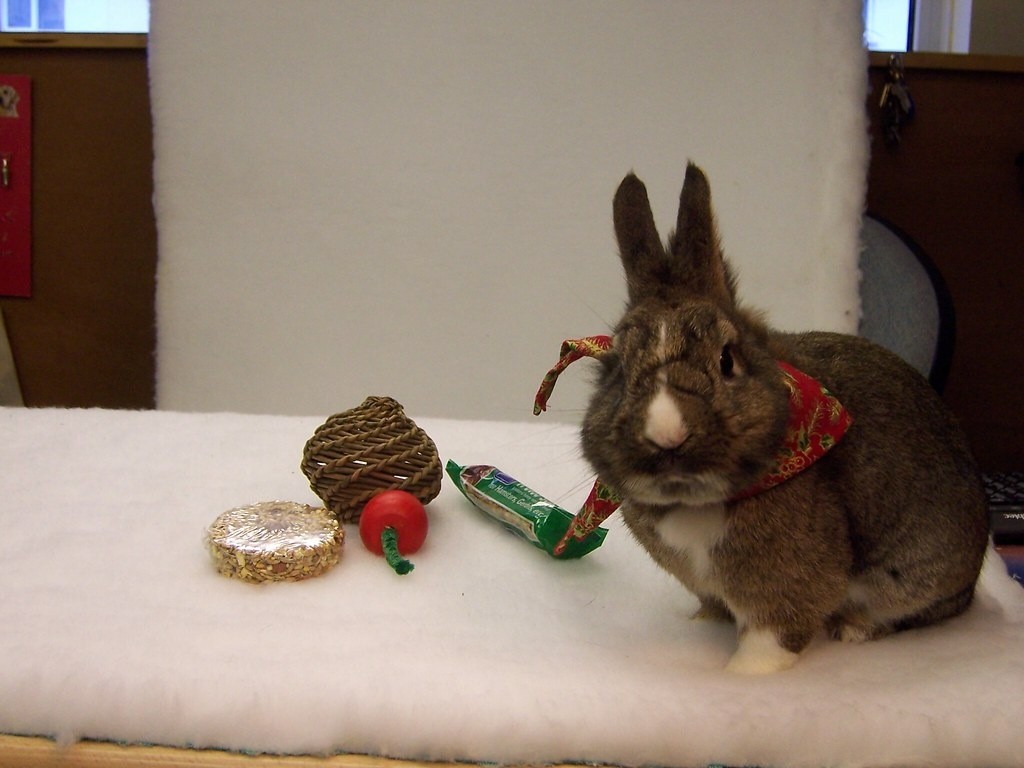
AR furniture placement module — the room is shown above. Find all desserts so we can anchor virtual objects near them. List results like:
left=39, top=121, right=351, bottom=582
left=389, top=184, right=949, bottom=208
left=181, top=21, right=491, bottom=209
left=205, top=501, right=345, bottom=584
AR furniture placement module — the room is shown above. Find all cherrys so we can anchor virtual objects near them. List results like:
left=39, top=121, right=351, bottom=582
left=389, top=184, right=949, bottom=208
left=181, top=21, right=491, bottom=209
left=359, top=490, right=428, bottom=575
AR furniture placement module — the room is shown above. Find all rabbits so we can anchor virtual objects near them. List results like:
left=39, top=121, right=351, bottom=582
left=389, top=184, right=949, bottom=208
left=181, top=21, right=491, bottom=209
left=579, top=159, right=989, bottom=677
